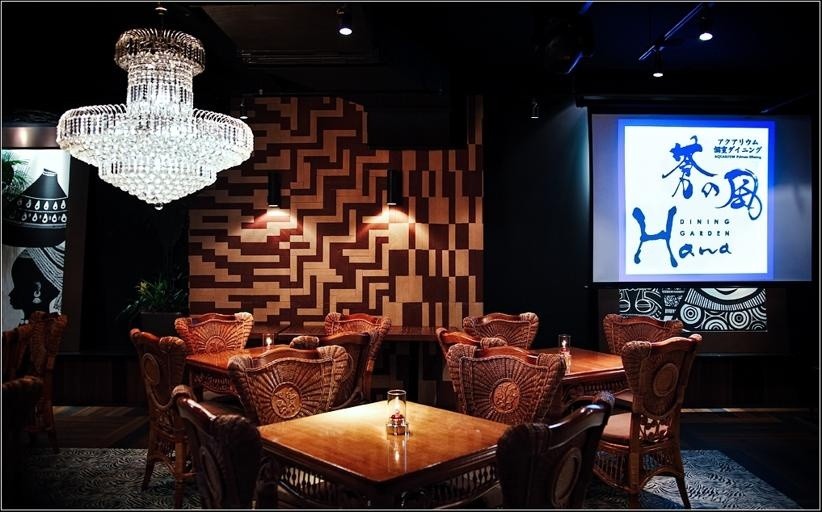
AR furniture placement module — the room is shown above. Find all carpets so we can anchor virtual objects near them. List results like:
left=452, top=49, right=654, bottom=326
left=29, top=446, right=804, bottom=509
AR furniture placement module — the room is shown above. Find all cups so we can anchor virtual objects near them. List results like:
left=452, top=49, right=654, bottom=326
left=386, top=390, right=407, bottom=435
left=387, top=437, right=406, bottom=476
left=263, top=334, right=274, bottom=350
left=558, top=334, right=571, bottom=357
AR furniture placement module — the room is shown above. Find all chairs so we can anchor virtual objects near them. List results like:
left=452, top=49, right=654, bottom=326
left=3, top=309, right=68, bottom=508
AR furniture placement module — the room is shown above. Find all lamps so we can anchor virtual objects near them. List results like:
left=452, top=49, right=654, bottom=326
left=266, top=172, right=280, bottom=207
left=698, top=13, right=714, bottom=42
left=649, top=54, right=664, bottom=78
left=529, top=101, right=540, bottom=119
left=335, top=7, right=353, bottom=36
left=387, top=170, right=398, bottom=206
left=239, top=105, right=248, bottom=120
left=154, top=3, right=168, bottom=15
left=55, top=1, right=255, bottom=210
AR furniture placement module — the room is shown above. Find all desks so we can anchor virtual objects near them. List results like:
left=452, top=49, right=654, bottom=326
left=245, top=323, right=456, bottom=412
left=256, top=399, right=513, bottom=510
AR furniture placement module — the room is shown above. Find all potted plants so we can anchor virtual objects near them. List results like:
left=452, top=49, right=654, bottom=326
left=118, top=270, right=188, bottom=336
